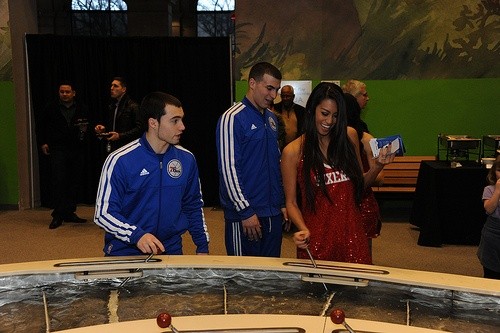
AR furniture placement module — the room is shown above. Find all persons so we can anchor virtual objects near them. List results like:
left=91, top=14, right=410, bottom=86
left=476, top=156, right=500, bottom=280
left=42, top=84, right=92, bottom=229
left=217, top=61, right=288, bottom=257
left=94, top=92, right=210, bottom=257
left=341, top=79, right=381, bottom=186
left=95, top=78, right=139, bottom=152
left=280, top=82, right=395, bottom=264
left=268, top=85, right=308, bottom=151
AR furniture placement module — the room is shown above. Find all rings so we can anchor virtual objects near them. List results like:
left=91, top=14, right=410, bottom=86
left=382, top=156, right=386, bottom=159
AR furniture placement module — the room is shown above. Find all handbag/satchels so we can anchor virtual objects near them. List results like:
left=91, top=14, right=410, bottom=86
left=359, top=184, right=382, bottom=239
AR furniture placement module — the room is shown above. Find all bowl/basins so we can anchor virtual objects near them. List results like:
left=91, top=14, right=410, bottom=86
left=481, top=158, right=496, bottom=164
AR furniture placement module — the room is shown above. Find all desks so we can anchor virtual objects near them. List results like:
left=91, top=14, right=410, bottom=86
left=408, top=158, right=491, bottom=245
left=438, top=134, right=483, bottom=168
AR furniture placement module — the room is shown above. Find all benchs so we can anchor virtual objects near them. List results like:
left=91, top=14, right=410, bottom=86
left=371, top=155, right=436, bottom=193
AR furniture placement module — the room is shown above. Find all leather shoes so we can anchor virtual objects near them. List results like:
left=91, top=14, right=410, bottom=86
left=63, top=212, right=87, bottom=223
left=49, top=214, right=64, bottom=228
